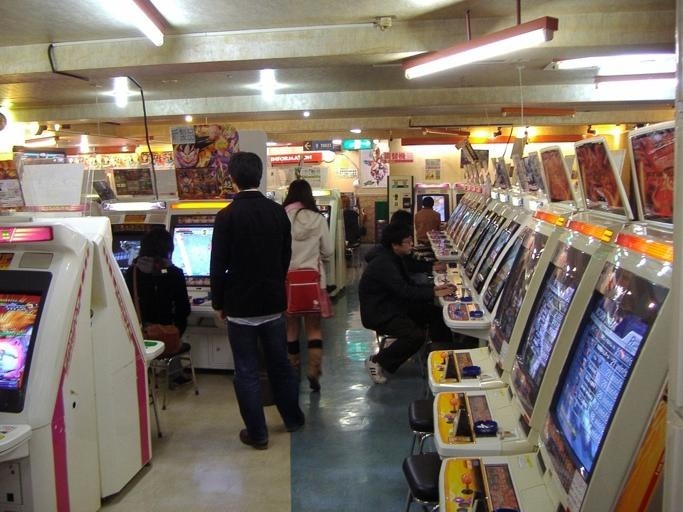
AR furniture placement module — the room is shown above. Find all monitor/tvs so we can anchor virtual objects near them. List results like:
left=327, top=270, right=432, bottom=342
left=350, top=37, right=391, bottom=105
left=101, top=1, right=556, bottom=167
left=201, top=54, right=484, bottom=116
left=446, top=199, right=529, bottom=315
left=509, top=240, right=591, bottom=416
left=171, top=224, right=214, bottom=278
left=421, top=195, right=446, bottom=221
left=0, top=288, right=43, bottom=391
left=548, top=261, right=670, bottom=484
left=487, top=224, right=549, bottom=354
left=322, top=212, right=329, bottom=220
left=112, top=238, right=142, bottom=275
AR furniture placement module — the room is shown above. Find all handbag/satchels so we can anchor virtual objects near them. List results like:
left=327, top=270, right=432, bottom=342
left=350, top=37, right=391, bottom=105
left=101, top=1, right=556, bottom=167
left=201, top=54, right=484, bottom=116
left=233, top=367, right=278, bottom=407
left=142, top=323, right=183, bottom=358
left=285, top=255, right=321, bottom=316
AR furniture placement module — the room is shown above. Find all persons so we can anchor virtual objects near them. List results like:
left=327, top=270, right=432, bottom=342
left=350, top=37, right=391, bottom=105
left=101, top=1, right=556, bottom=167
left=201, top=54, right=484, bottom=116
left=208, top=151, right=306, bottom=450
left=364, top=209, right=452, bottom=342
left=357, top=220, right=454, bottom=384
left=342, top=195, right=360, bottom=214
left=414, top=196, right=441, bottom=244
left=282, top=179, right=334, bottom=391
left=123, top=227, right=193, bottom=391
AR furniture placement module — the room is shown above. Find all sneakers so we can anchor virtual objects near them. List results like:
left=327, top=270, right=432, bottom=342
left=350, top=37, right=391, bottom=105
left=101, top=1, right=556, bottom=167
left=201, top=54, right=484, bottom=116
left=170, top=372, right=196, bottom=390
left=284, top=414, right=304, bottom=431
left=240, top=428, right=269, bottom=450
left=365, top=355, right=387, bottom=385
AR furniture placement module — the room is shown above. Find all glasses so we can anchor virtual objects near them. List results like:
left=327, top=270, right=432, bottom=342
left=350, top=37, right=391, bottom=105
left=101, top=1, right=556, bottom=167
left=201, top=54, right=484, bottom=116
left=402, top=240, right=415, bottom=243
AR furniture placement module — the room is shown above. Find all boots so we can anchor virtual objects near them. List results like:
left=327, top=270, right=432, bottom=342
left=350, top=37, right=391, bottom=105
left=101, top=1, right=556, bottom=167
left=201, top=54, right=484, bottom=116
left=307, top=346, right=321, bottom=389
left=289, top=353, right=301, bottom=389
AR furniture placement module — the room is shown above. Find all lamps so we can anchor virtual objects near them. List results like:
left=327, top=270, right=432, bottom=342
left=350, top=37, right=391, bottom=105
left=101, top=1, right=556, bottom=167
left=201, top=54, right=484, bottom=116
left=400, top=0, right=559, bottom=82
left=592, top=74, right=678, bottom=93
left=422, top=127, right=473, bottom=141
left=118, top=0, right=174, bottom=48
left=501, top=107, right=579, bottom=120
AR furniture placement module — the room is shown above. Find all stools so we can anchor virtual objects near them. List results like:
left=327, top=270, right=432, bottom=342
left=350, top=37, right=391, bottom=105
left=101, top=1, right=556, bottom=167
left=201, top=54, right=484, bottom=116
left=380, top=244, right=443, bottom=512
left=153, top=342, right=200, bottom=409
left=345, top=241, right=362, bottom=266
